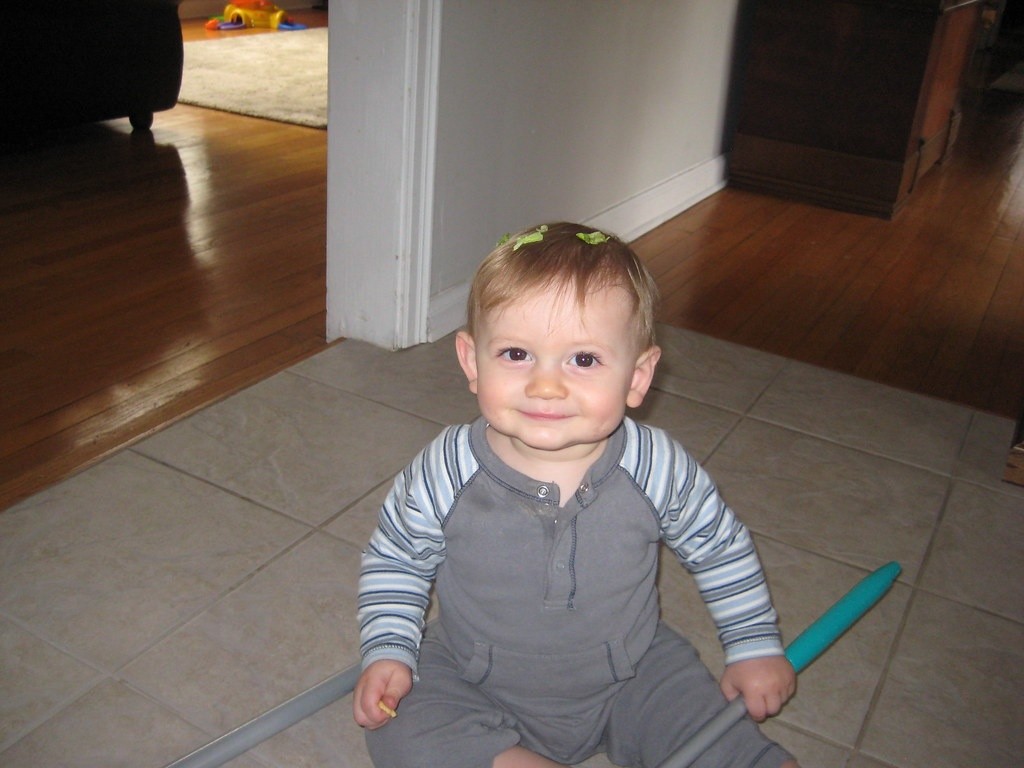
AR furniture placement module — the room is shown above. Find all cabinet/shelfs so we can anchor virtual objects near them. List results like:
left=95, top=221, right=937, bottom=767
left=719, top=0, right=997, bottom=223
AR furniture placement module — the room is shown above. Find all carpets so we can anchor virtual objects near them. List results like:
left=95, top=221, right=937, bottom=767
left=177, top=24, right=329, bottom=131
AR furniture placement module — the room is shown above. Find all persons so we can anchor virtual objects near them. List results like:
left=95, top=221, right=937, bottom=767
left=354, top=223, right=802, bottom=768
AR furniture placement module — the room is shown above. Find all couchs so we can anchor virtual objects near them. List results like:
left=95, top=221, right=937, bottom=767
left=0, top=0, right=185, bottom=139
